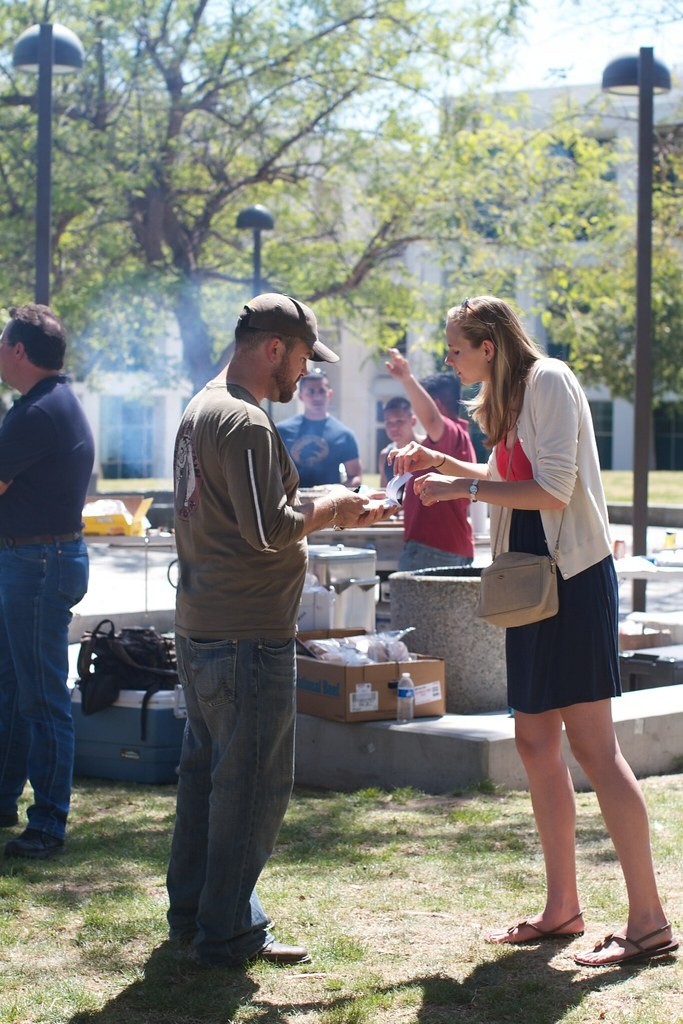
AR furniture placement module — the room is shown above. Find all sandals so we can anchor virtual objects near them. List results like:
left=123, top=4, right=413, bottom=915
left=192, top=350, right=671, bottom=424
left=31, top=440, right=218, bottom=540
left=484, top=912, right=584, bottom=944
left=575, top=922, right=680, bottom=965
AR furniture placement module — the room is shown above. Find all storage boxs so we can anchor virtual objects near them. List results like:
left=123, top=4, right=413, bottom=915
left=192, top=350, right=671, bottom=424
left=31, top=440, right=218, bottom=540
left=294, top=629, right=447, bottom=724
left=81, top=494, right=154, bottom=538
left=618, top=644, right=683, bottom=693
left=296, top=585, right=335, bottom=632
left=307, top=544, right=378, bottom=588
left=324, top=576, right=380, bottom=633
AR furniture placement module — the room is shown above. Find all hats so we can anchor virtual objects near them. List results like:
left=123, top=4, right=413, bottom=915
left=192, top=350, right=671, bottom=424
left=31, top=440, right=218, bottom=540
left=238, top=293, right=338, bottom=363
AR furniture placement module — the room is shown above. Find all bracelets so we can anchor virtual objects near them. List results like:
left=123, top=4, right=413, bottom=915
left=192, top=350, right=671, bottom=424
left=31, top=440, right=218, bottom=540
left=432, top=452, right=445, bottom=468
left=332, top=524, right=344, bottom=531
left=329, top=498, right=338, bottom=524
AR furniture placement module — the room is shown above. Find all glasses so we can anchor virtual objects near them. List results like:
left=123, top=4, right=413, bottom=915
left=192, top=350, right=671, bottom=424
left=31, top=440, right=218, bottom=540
left=462, top=298, right=484, bottom=321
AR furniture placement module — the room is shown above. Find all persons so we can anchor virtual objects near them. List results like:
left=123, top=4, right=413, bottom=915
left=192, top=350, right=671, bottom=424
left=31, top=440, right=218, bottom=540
left=388, top=297, right=680, bottom=966
left=0, top=301, right=95, bottom=860
left=272, top=368, right=361, bottom=490
left=378, top=348, right=478, bottom=571
left=167, top=294, right=400, bottom=967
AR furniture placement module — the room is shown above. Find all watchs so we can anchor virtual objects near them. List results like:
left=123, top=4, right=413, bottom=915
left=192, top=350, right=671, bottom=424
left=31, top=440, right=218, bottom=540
left=468, top=479, right=479, bottom=503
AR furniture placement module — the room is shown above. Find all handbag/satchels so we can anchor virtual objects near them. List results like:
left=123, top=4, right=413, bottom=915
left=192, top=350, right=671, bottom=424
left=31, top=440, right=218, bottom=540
left=77, top=619, right=177, bottom=741
left=479, top=552, right=558, bottom=628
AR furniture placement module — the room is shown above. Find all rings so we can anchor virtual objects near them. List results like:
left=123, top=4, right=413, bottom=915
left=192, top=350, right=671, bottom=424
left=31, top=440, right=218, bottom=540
left=420, top=489, right=427, bottom=497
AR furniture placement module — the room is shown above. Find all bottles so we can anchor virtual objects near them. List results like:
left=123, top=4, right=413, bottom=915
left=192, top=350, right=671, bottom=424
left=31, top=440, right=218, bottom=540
left=396, top=672, right=414, bottom=724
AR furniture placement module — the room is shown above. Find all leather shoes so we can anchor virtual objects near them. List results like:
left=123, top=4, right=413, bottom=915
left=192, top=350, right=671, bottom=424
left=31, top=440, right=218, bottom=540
left=263, top=914, right=275, bottom=932
left=5, top=828, right=64, bottom=860
left=200, top=939, right=311, bottom=965
left=0, top=810, right=18, bottom=827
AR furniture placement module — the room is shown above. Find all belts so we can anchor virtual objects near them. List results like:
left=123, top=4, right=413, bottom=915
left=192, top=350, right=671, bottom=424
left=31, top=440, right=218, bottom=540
left=2, top=532, right=79, bottom=549
